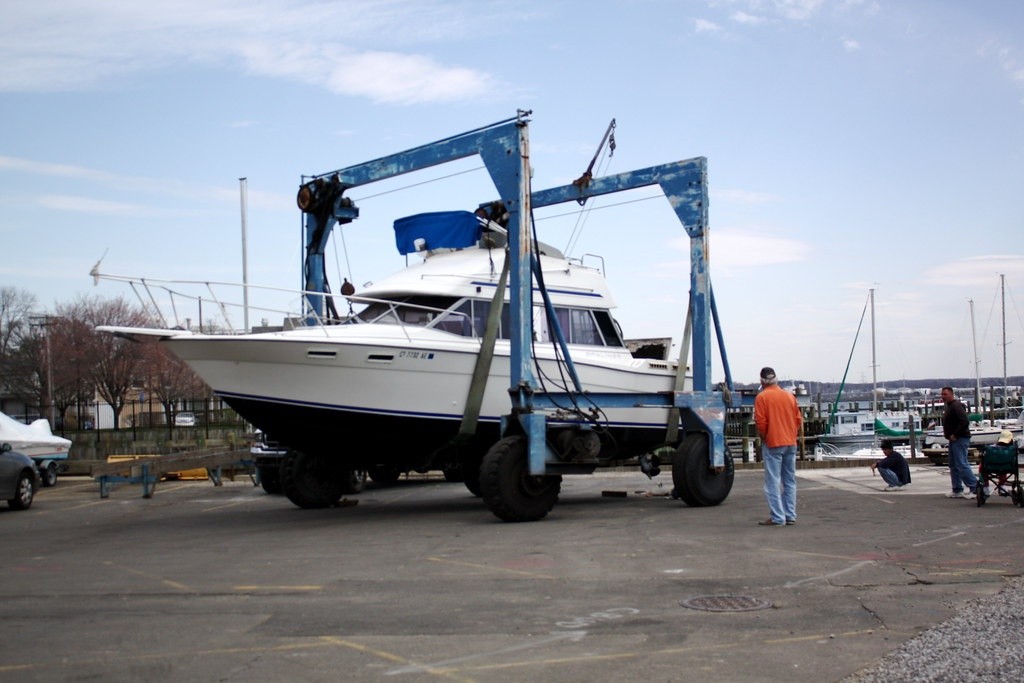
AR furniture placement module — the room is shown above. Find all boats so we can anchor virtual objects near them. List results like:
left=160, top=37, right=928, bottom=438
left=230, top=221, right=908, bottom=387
left=95, top=209, right=688, bottom=474
left=0, top=410, right=72, bottom=462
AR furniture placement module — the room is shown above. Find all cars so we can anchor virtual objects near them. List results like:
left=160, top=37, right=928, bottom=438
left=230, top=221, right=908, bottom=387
left=173, top=411, right=195, bottom=426
left=0, top=442, right=43, bottom=510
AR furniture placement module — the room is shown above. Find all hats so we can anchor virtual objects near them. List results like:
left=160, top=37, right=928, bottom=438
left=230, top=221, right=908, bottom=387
left=761, top=367, right=776, bottom=379
left=998, top=430, right=1013, bottom=444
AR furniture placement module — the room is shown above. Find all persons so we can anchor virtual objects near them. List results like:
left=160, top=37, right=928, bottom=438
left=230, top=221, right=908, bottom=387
left=754, top=366, right=803, bottom=527
left=978, top=430, right=1017, bottom=496
left=941, top=387, right=980, bottom=500
left=871, top=439, right=912, bottom=492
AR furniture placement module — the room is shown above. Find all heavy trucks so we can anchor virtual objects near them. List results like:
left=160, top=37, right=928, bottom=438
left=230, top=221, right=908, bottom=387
left=249, top=424, right=461, bottom=497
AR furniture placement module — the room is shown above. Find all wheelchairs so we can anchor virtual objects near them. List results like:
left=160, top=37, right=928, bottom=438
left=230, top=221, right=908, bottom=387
left=975, top=444, right=1024, bottom=508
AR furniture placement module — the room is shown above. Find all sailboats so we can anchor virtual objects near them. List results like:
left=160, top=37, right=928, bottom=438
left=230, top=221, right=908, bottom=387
left=817, top=273, right=1024, bottom=464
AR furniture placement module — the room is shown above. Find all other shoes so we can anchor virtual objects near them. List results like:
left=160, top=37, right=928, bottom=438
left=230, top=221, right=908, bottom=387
left=945, top=489, right=964, bottom=497
left=999, top=485, right=1009, bottom=493
left=963, top=491, right=977, bottom=500
left=758, top=519, right=782, bottom=526
left=983, top=486, right=992, bottom=496
left=785, top=519, right=796, bottom=525
left=885, top=485, right=904, bottom=491
